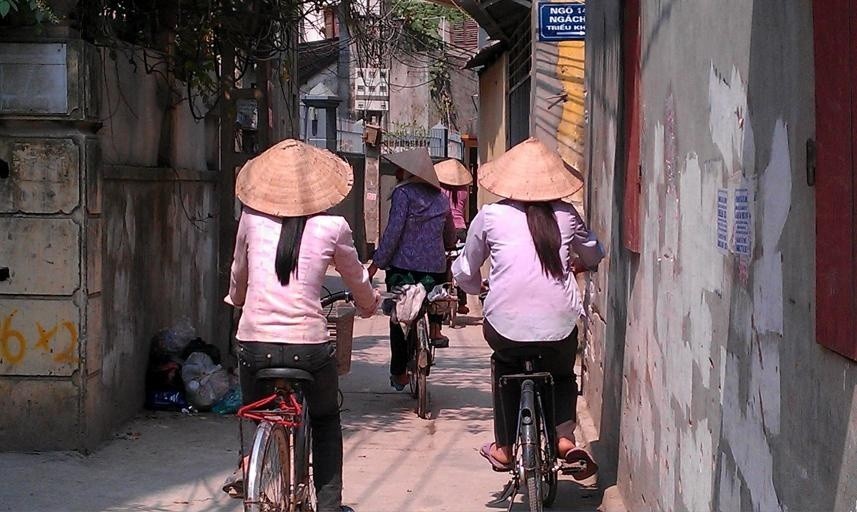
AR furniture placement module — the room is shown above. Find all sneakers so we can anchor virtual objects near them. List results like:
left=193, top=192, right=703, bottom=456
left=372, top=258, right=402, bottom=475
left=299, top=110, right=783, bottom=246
left=222, top=470, right=245, bottom=493
left=458, top=306, right=469, bottom=313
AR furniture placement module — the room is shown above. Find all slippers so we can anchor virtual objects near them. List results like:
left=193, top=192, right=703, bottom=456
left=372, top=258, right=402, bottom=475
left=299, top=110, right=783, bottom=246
left=390, top=375, right=404, bottom=391
left=480, top=441, right=513, bottom=471
left=432, top=335, right=448, bottom=347
left=565, top=447, right=598, bottom=481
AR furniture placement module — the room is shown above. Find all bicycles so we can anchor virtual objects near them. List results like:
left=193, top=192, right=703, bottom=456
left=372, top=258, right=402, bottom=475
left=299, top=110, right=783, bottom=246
left=390, top=298, right=449, bottom=420
left=445, top=246, right=466, bottom=327
left=453, top=264, right=597, bottom=511
left=231, top=285, right=354, bottom=512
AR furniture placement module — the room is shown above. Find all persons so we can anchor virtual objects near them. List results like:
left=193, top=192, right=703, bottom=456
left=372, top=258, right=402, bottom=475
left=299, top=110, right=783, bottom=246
left=440, top=183, right=471, bottom=314
left=362, top=166, right=457, bottom=391
left=449, top=199, right=605, bottom=482
left=221, top=200, right=383, bottom=511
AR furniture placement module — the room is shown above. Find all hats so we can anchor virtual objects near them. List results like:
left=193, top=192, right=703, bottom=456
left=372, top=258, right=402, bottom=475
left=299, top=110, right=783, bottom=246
left=434, top=159, right=474, bottom=186
left=236, top=138, right=354, bottom=218
left=383, top=147, right=441, bottom=189
left=476, top=139, right=584, bottom=202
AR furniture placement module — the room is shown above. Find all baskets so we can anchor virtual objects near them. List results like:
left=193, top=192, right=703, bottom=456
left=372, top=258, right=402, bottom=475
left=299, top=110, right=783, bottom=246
left=321, top=298, right=355, bottom=375
